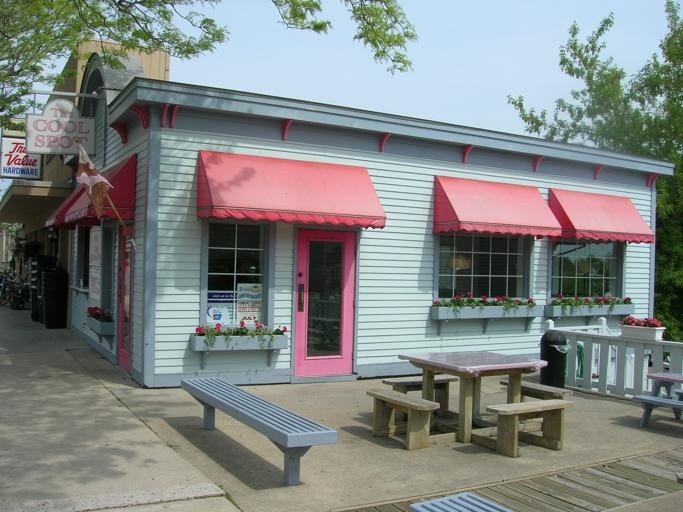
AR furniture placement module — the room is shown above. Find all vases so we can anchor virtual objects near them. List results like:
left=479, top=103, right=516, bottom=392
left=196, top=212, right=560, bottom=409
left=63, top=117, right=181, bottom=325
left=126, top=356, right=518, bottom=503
left=189, top=334, right=288, bottom=372
left=544, top=305, right=635, bottom=327
left=618, top=323, right=666, bottom=340
left=428, top=306, right=539, bottom=335
left=84, top=315, right=115, bottom=336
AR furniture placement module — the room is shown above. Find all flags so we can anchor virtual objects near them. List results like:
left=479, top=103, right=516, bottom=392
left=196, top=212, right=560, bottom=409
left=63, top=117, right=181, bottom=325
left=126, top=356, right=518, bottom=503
left=75, top=145, right=111, bottom=220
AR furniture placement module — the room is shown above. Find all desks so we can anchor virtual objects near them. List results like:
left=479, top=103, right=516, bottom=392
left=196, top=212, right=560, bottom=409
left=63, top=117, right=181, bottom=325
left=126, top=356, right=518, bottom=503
left=639, top=373, right=683, bottom=427
left=397, top=350, right=549, bottom=444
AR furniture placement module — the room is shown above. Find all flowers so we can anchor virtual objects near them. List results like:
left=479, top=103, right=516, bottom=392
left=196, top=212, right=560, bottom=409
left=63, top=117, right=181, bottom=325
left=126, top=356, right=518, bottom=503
left=548, top=293, right=631, bottom=313
left=86, top=306, right=112, bottom=322
left=433, top=291, right=536, bottom=316
left=619, top=314, right=662, bottom=327
left=196, top=319, right=288, bottom=349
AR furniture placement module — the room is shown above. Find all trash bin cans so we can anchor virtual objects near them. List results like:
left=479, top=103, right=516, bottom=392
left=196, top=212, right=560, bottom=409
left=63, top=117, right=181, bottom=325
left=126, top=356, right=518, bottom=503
left=540, top=330, right=568, bottom=387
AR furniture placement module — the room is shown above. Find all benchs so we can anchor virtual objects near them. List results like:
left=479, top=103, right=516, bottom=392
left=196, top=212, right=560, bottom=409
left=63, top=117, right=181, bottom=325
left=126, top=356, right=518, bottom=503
left=382, top=374, right=459, bottom=423
left=499, top=378, right=573, bottom=402
left=486, top=398, right=575, bottom=457
left=411, top=492, right=507, bottom=511
left=180, top=376, right=339, bottom=487
left=673, top=389, right=683, bottom=400
left=632, top=395, right=683, bottom=412
left=365, top=388, right=440, bottom=451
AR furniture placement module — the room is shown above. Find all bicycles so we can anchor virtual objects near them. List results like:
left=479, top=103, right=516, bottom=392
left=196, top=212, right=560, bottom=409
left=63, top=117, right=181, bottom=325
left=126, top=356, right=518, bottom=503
left=0, top=271, right=29, bottom=309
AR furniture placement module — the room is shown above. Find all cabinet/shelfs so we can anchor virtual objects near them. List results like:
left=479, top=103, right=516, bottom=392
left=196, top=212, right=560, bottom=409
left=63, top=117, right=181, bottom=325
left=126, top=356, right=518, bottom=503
left=29, top=257, right=68, bottom=329
